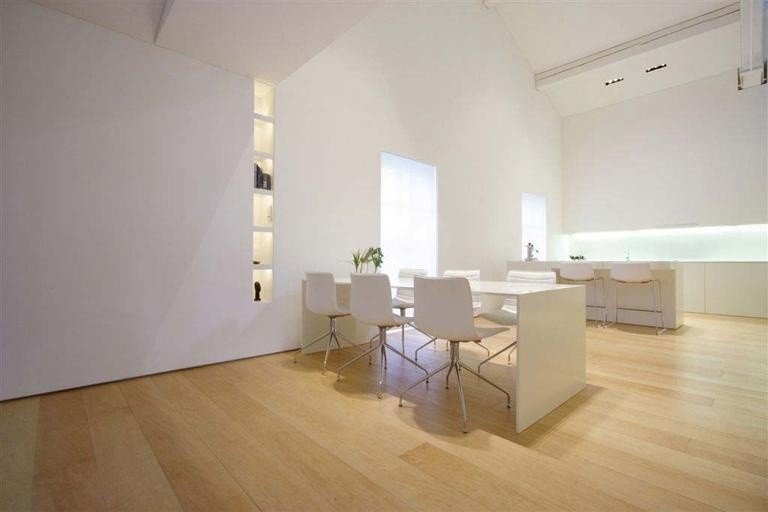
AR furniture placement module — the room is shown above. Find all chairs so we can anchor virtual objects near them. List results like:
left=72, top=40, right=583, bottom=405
left=295, top=272, right=372, bottom=376
left=477, top=269, right=557, bottom=374
left=398, top=275, right=511, bottom=433
left=557, top=260, right=667, bottom=335
left=369, top=269, right=436, bottom=350
left=415, top=270, right=489, bottom=361
left=336, top=272, right=431, bottom=383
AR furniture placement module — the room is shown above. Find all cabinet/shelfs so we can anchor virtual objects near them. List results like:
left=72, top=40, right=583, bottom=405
left=250, top=79, right=277, bottom=306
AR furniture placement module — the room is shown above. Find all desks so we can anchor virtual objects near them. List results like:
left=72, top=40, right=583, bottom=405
left=333, top=270, right=557, bottom=434
